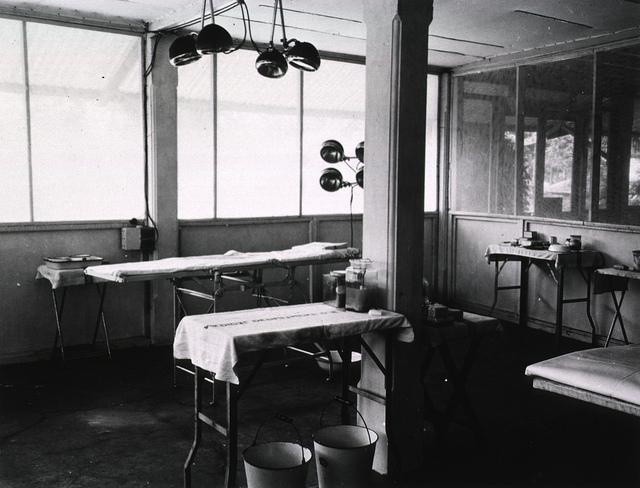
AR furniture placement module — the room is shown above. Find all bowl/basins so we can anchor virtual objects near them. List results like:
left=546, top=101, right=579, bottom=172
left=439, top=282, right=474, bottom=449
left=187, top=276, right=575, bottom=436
left=313, top=349, right=361, bottom=374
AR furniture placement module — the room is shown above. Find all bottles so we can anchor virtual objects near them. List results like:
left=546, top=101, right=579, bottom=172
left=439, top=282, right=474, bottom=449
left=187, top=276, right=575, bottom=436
left=330, top=269, right=347, bottom=308
left=548, top=234, right=582, bottom=251
left=632, top=249, right=640, bottom=272
left=421, top=275, right=430, bottom=304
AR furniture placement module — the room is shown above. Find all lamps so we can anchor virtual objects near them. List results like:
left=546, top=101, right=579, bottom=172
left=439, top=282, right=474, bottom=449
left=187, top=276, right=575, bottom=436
left=171, top=0, right=246, bottom=66
left=317, top=137, right=364, bottom=247
left=241, top=0, right=319, bottom=79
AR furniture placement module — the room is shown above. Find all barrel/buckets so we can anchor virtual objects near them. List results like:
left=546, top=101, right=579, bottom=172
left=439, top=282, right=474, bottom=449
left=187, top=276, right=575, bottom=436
left=311, top=398, right=379, bottom=488
left=242, top=416, right=313, bottom=488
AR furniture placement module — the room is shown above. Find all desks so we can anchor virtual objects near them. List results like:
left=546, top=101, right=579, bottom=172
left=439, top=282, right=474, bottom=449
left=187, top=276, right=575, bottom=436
left=594, top=264, right=639, bottom=348
left=174, top=298, right=416, bottom=487
left=83, top=242, right=366, bottom=406
left=524, top=342, right=640, bottom=419
left=36, top=264, right=115, bottom=362
left=422, top=300, right=497, bottom=455
left=489, top=245, right=597, bottom=356
